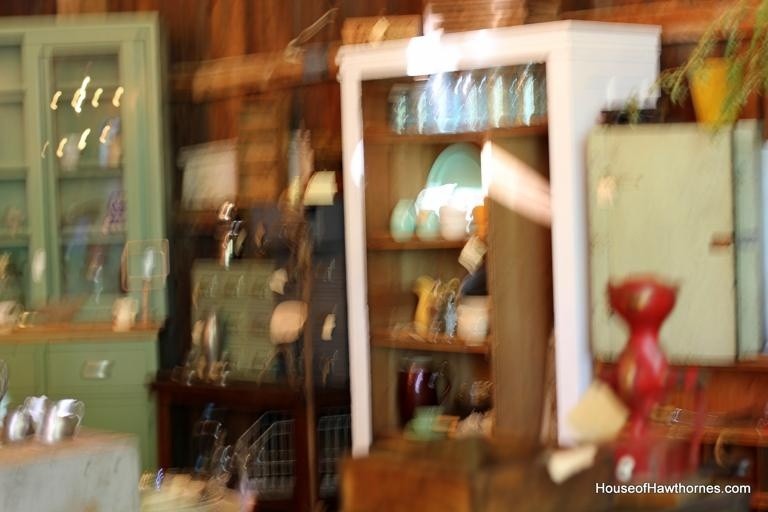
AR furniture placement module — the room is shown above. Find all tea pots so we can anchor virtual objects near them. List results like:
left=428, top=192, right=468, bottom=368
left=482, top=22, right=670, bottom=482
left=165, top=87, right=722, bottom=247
left=396, top=356, right=451, bottom=424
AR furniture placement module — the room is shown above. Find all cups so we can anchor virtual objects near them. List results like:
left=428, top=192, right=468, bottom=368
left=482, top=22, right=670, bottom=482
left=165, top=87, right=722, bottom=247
left=438, top=207, right=467, bottom=243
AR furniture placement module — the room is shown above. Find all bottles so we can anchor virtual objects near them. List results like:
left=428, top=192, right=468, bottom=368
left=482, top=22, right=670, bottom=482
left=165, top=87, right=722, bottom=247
left=415, top=211, right=441, bottom=242
left=388, top=66, right=547, bottom=136
left=387, top=198, right=417, bottom=243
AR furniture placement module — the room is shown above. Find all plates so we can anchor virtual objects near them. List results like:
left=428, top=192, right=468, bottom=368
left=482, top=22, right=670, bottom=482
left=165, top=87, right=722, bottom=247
left=426, top=141, right=485, bottom=192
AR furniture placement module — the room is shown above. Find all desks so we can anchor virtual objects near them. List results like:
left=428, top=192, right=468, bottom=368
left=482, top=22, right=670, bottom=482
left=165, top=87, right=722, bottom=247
left=0, top=431, right=142, bottom=512
left=147, top=366, right=350, bottom=512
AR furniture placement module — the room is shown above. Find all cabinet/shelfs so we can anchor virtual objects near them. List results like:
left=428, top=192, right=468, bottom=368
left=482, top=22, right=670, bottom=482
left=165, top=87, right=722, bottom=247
left=0, top=11, right=174, bottom=473
left=337, top=21, right=662, bottom=457
